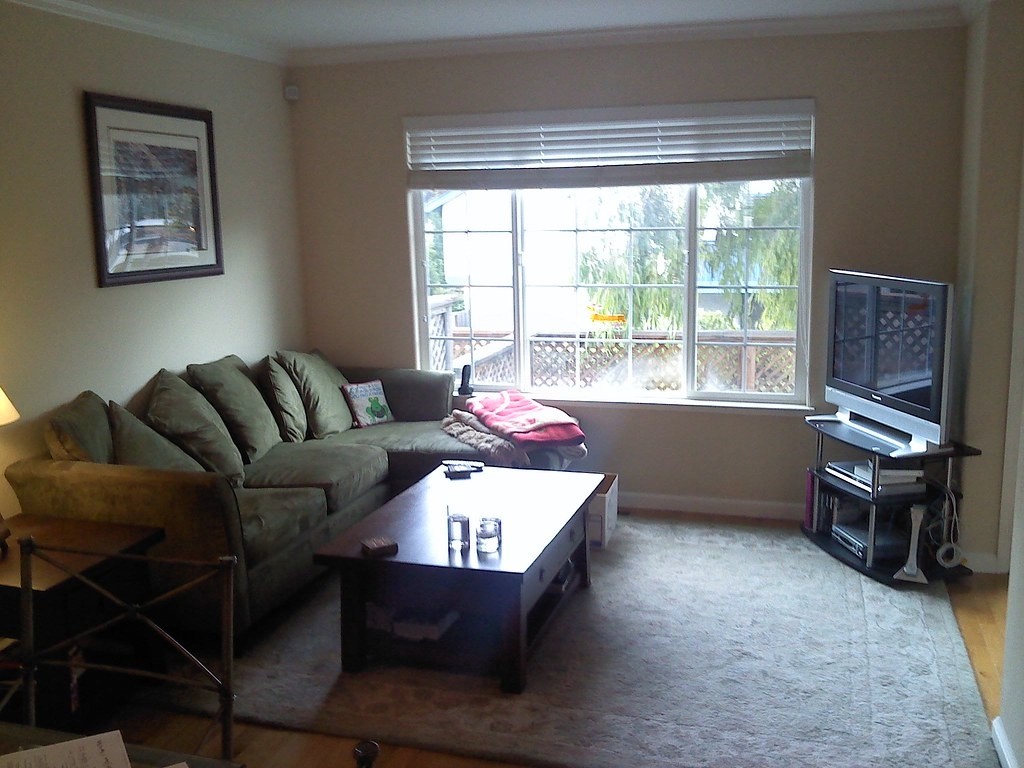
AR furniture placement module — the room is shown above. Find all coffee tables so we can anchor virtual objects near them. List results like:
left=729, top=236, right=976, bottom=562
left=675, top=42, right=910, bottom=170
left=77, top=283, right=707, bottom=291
left=312, top=464, right=605, bottom=696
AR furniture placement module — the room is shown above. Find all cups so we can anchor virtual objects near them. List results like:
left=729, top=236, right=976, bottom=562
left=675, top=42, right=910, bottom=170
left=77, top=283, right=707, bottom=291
left=447, top=503, right=470, bottom=550
left=476, top=518, right=502, bottom=553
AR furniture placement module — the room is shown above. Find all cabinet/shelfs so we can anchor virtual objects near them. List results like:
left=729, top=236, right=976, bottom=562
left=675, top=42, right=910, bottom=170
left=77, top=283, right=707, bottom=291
left=802, top=416, right=982, bottom=589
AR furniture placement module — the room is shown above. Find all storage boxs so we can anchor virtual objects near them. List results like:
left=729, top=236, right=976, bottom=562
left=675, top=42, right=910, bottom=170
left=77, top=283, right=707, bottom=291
left=588, top=472, right=618, bottom=551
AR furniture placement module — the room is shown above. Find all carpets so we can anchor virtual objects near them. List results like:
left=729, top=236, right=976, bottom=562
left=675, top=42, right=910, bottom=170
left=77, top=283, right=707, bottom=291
left=50, top=509, right=1001, bottom=768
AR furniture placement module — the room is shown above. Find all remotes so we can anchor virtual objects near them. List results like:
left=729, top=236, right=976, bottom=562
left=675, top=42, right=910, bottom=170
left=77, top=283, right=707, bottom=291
left=442, top=460, right=484, bottom=468
left=448, top=465, right=478, bottom=473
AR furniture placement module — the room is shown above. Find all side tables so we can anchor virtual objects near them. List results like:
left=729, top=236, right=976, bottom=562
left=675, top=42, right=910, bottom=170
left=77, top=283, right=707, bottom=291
left=0, top=513, right=166, bottom=734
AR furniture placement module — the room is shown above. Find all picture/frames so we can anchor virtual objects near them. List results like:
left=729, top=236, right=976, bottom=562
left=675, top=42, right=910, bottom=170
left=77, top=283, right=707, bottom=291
left=81, top=88, right=225, bottom=288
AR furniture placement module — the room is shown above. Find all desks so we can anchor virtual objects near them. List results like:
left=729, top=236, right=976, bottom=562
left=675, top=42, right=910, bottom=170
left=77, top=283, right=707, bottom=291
left=0, top=722, right=246, bottom=768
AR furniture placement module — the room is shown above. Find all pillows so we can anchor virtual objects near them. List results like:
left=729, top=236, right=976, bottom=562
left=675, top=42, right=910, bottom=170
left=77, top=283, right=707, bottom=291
left=275, top=346, right=353, bottom=440
left=144, top=368, right=246, bottom=489
left=186, top=353, right=283, bottom=464
left=108, top=399, right=206, bottom=472
left=264, top=355, right=308, bottom=443
left=41, top=390, right=115, bottom=464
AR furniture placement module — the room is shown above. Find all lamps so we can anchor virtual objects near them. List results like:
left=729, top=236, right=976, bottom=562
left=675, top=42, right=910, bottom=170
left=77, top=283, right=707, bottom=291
left=0, top=386, right=22, bottom=561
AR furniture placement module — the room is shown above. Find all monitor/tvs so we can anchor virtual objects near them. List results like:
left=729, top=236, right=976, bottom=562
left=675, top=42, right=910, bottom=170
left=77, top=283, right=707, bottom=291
left=806, top=267, right=955, bottom=457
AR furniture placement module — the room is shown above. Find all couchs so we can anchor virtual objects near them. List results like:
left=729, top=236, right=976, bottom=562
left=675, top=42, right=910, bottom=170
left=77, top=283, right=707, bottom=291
left=5, top=367, right=540, bottom=656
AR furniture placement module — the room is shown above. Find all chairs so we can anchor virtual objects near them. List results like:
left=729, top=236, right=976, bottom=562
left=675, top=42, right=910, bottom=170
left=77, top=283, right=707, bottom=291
left=15, top=535, right=238, bottom=763
